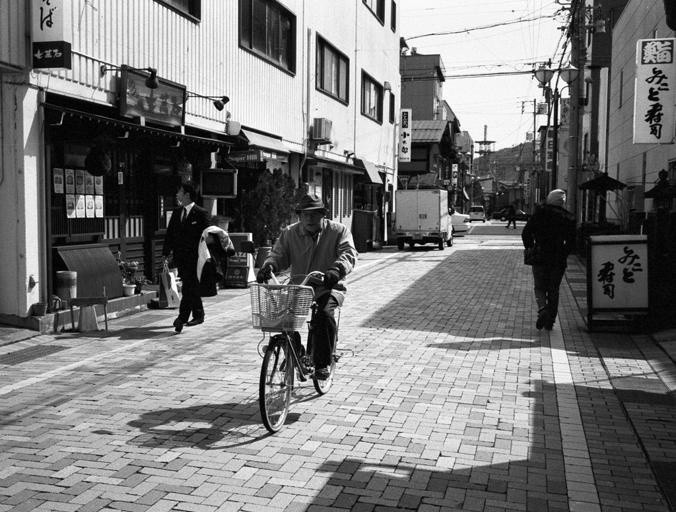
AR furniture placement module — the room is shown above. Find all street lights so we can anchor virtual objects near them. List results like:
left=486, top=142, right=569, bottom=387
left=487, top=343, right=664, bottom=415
left=533, top=63, right=581, bottom=190
left=455, top=151, right=471, bottom=214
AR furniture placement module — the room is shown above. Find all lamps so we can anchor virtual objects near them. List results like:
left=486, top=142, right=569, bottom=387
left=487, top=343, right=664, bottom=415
left=101, top=65, right=159, bottom=89
left=186, top=92, right=229, bottom=111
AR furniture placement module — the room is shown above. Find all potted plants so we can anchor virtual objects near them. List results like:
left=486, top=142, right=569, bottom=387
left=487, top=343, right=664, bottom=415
left=133, top=276, right=152, bottom=294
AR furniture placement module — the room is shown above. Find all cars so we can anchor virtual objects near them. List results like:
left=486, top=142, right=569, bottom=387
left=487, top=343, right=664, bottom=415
left=449, top=208, right=471, bottom=236
left=491, top=208, right=528, bottom=222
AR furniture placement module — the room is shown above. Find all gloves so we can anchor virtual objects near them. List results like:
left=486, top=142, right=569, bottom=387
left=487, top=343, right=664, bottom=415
left=256, top=265, right=272, bottom=284
left=325, top=269, right=340, bottom=285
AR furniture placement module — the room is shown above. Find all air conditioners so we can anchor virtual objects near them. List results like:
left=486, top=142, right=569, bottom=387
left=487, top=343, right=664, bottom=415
left=312, top=118, right=333, bottom=141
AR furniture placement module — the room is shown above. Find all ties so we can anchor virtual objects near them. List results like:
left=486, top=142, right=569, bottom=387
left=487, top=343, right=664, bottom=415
left=182, top=207, right=187, bottom=226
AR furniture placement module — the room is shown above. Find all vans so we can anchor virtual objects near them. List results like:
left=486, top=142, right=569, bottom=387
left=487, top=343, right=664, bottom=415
left=469, top=206, right=485, bottom=222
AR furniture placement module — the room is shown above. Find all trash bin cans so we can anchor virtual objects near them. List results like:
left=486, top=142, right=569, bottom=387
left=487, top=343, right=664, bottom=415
left=353, top=209, right=377, bottom=253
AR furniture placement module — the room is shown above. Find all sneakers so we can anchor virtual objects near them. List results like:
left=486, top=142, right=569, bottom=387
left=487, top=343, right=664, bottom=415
left=536, top=309, right=553, bottom=330
left=315, top=363, right=332, bottom=378
left=279, top=345, right=305, bottom=372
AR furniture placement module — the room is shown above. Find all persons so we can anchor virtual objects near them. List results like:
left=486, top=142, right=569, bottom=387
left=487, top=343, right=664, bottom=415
left=161, top=184, right=214, bottom=332
left=522, top=189, right=576, bottom=330
left=258, top=194, right=356, bottom=379
left=505, top=204, right=517, bottom=229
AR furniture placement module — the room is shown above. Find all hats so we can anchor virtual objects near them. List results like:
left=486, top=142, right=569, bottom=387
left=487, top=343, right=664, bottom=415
left=295, top=195, right=329, bottom=215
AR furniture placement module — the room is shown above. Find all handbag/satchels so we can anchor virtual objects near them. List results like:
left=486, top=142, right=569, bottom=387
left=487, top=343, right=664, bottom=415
left=159, top=271, right=180, bottom=308
left=523, top=248, right=538, bottom=265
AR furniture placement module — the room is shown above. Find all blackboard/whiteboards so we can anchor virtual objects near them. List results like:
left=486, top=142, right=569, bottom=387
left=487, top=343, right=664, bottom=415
left=200, top=168, right=238, bottom=198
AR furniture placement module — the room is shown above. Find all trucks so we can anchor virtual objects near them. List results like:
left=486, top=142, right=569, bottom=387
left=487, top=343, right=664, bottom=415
left=392, top=188, right=454, bottom=252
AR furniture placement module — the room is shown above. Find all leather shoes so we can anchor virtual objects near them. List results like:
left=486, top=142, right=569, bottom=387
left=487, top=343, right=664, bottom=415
left=172, top=313, right=204, bottom=332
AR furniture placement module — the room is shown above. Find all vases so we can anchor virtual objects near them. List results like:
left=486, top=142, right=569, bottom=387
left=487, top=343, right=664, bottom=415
left=123, top=284, right=137, bottom=295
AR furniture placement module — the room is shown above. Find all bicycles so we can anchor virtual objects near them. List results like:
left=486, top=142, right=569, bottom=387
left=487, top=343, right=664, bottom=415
left=249, top=266, right=342, bottom=434
